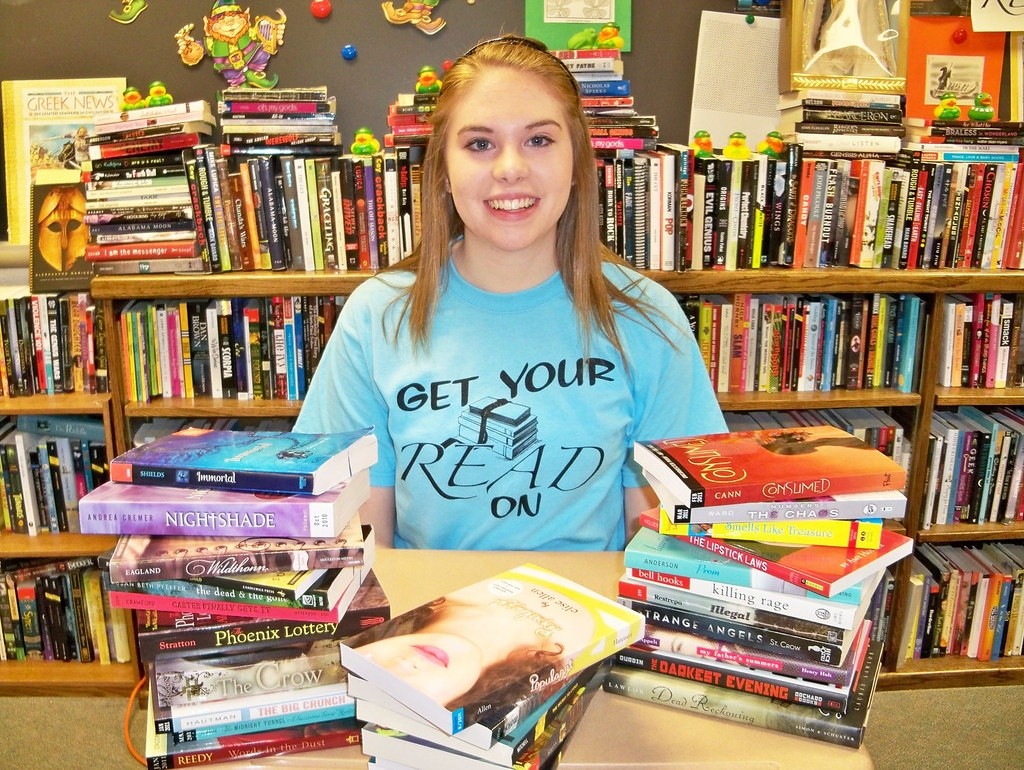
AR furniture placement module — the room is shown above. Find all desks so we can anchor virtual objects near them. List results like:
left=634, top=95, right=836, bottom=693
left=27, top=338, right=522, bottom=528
left=0, top=683, right=1024, bottom=770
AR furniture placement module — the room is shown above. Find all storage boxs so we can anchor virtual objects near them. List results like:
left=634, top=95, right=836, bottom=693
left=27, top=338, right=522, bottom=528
left=778, top=0, right=911, bottom=95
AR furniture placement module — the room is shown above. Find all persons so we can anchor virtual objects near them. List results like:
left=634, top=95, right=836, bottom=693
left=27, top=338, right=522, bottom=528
left=292, top=32, right=725, bottom=552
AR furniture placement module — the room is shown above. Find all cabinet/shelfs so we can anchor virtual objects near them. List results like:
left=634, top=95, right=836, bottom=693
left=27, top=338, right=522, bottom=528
left=0, top=262, right=1024, bottom=701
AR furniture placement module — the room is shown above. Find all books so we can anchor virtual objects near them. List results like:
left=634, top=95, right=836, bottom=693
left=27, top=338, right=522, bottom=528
left=683, top=292, right=1024, bottom=392
left=1, top=47, right=1024, bottom=293
left=604, top=408, right=1024, bottom=750
left=0, top=295, right=344, bottom=401
left=340, top=563, right=646, bottom=769
left=0, top=416, right=392, bottom=769
